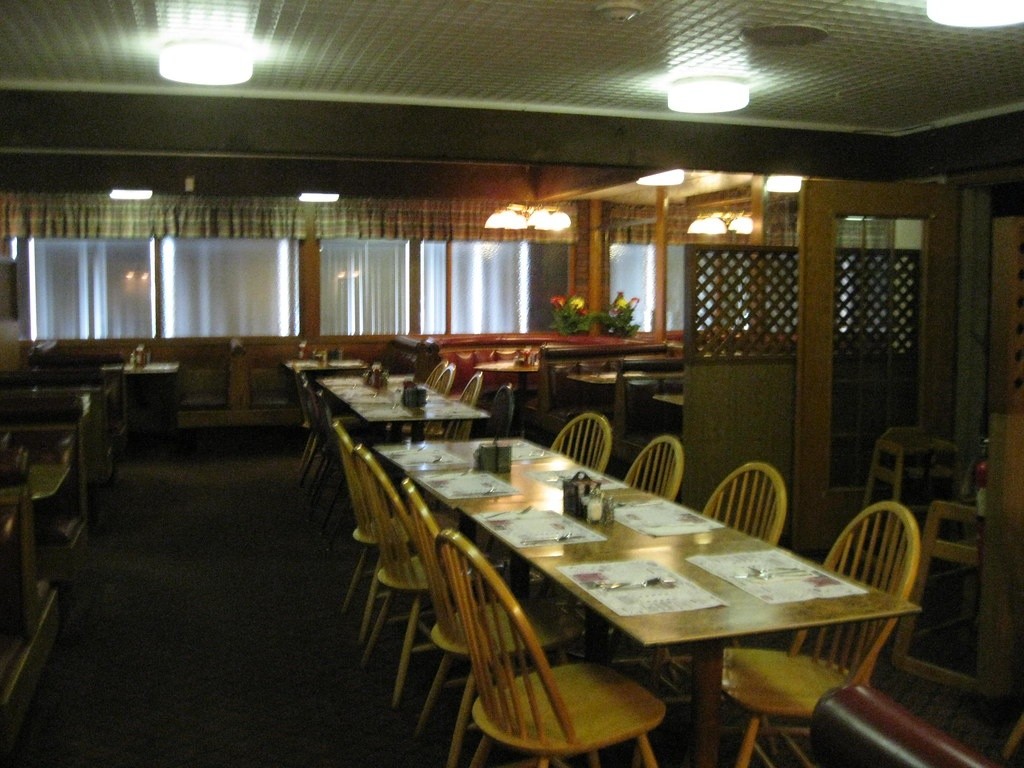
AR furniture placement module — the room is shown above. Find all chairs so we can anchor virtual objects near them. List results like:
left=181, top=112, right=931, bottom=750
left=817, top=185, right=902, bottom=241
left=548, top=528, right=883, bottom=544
left=291, top=358, right=922, bottom=768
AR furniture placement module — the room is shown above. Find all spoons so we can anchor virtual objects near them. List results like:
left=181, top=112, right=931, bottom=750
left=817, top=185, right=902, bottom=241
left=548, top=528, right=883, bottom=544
left=520, top=531, right=573, bottom=545
left=746, top=565, right=805, bottom=574
left=407, top=457, right=443, bottom=465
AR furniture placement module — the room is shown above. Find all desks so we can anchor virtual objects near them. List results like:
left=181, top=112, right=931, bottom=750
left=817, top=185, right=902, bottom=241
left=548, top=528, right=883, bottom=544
left=124, top=361, right=180, bottom=441
left=473, top=361, right=539, bottom=437
left=455, top=492, right=923, bottom=768
left=279, top=359, right=369, bottom=377
left=316, top=375, right=490, bottom=443
left=652, top=393, right=684, bottom=409
left=372, top=437, right=630, bottom=546
left=567, top=373, right=616, bottom=415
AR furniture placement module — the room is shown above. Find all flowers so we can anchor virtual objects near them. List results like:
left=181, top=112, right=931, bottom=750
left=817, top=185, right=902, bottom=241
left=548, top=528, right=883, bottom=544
left=591, top=290, right=641, bottom=338
left=548, top=287, right=594, bottom=338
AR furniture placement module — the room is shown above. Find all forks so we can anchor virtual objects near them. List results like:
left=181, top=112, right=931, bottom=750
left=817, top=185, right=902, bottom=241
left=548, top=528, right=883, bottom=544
left=486, top=505, right=534, bottom=519
left=391, top=445, right=426, bottom=456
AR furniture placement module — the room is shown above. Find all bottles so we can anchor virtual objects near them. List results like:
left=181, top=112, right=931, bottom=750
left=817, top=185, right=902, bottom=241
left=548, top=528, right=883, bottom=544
left=602, top=496, right=614, bottom=526
left=587, top=489, right=601, bottom=525
left=135, top=348, right=145, bottom=368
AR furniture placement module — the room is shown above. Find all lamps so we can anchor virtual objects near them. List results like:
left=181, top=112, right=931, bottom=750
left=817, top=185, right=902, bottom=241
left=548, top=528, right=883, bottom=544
left=667, top=75, right=753, bottom=114
left=484, top=201, right=572, bottom=231
left=158, top=39, right=254, bottom=85
left=688, top=210, right=753, bottom=235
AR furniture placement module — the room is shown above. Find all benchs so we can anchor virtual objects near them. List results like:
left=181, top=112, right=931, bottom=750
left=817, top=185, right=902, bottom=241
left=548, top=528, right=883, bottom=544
left=424, top=333, right=648, bottom=441
left=80, top=352, right=128, bottom=461
left=546, top=353, right=667, bottom=436
left=0, top=429, right=87, bottom=600
left=624, top=379, right=684, bottom=464
